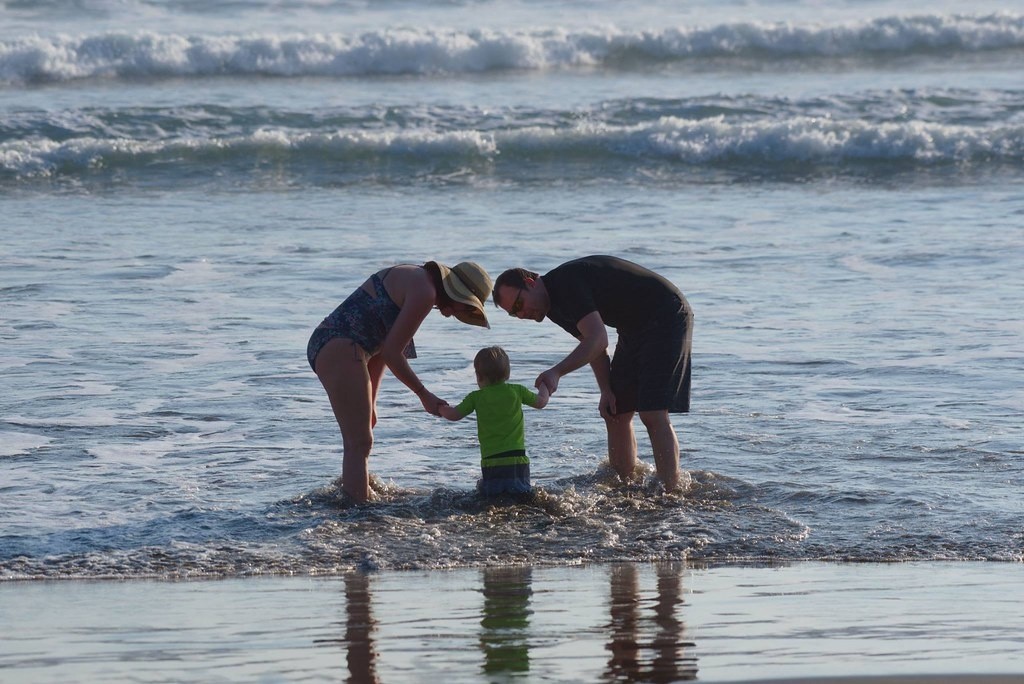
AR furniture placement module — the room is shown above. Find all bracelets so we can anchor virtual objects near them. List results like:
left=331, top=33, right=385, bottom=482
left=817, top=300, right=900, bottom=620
left=414, top=385, right=424, bottom=394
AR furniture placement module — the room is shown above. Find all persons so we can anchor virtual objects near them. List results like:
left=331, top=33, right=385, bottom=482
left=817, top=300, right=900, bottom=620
left=492, top=255, right=694, bottom=493
left=306, top=260, right=494, bottom=506
left=438, top=345, right=550, bottom=504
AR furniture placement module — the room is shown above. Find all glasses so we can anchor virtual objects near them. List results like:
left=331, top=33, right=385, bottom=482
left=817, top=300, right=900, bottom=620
left=509, top=283, right=526, bottom=318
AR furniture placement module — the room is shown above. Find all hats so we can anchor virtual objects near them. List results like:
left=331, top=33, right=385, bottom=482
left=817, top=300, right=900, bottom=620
left=423, top=260, right=494, bottom=329
